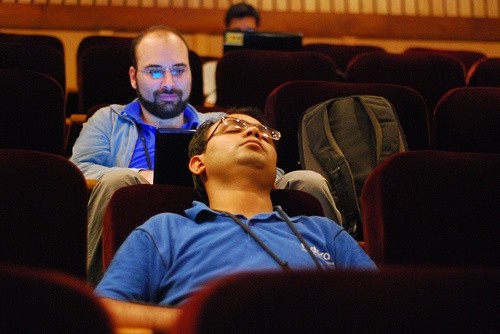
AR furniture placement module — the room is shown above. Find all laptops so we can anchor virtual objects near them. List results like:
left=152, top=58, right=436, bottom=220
left=153, top=127, right=197, bottom=187
left=223, top=29, right=303, bottom=53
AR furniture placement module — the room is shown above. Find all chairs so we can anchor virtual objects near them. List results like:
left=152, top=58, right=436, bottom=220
left=0, top=34, right=500, bottom=334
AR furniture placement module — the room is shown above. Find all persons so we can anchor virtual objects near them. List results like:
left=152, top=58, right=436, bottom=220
left=69, top=25, right=343, bottom=290
left=94, top=108, right=381, bottom=334
left=201, top=2, right=261, bottom=107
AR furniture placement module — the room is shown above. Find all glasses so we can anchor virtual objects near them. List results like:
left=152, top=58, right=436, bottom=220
left=135, top=67, right=187, bottom=79
left=204, top=118, right=281, bottom=141
